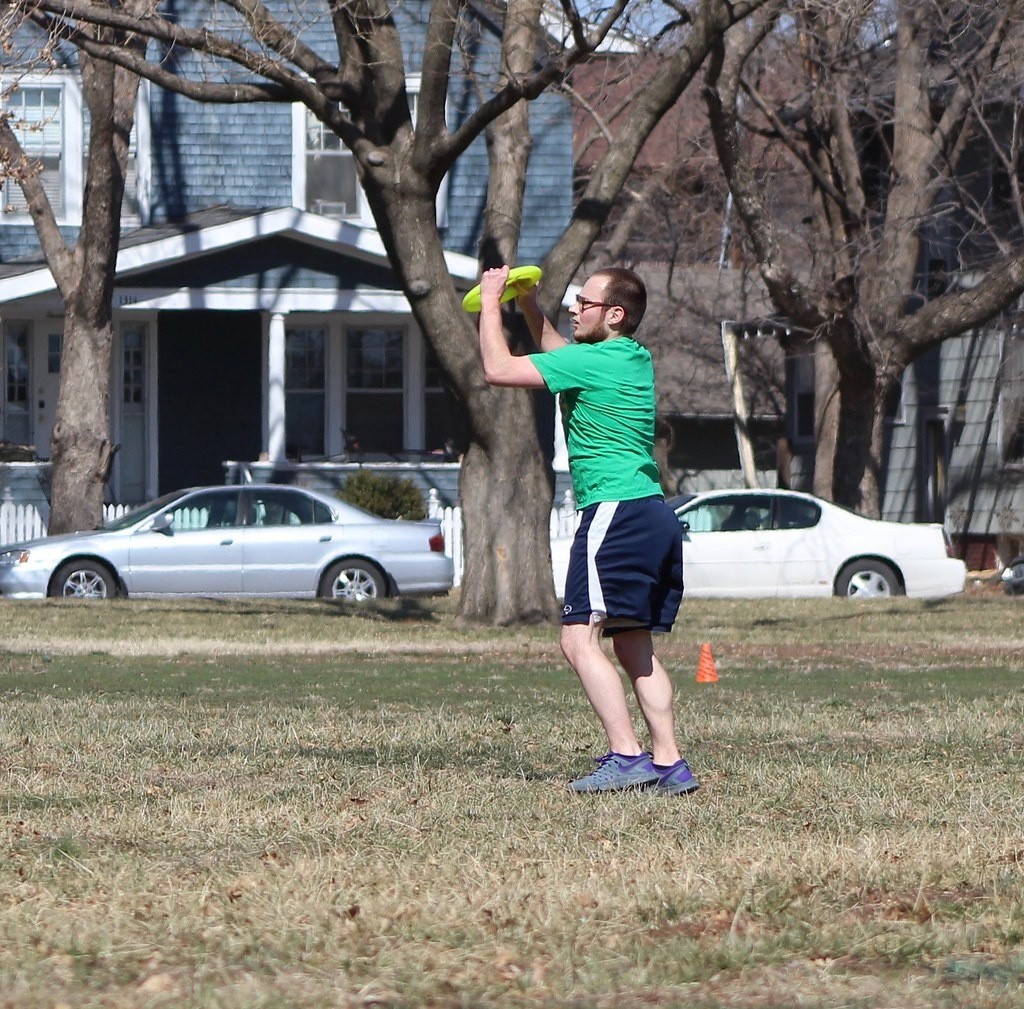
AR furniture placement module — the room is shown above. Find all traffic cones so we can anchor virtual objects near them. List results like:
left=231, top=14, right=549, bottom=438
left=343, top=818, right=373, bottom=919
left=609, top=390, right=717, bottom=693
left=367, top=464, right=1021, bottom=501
left=694, top=642, right=721, bottom=684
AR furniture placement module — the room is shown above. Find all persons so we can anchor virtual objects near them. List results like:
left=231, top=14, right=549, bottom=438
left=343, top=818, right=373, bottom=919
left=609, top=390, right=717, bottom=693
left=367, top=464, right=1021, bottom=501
left=480, top=265, right=700, bottom=796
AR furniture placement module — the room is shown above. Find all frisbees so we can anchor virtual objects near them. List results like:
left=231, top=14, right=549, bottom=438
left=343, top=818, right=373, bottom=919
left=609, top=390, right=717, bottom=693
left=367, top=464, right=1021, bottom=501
left=461, top=264, right=543, bottom=313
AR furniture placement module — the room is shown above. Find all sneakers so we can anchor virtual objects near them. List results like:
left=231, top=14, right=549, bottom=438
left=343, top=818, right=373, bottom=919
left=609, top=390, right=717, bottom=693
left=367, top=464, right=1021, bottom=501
left=639, top=759, right=699, bottom=797
left=567, top=748, right=659, bottom=792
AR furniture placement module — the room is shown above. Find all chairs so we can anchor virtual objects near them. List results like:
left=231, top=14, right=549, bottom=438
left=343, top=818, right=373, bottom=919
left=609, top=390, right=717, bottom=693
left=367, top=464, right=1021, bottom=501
left=738, top=510, right=759, bottom=529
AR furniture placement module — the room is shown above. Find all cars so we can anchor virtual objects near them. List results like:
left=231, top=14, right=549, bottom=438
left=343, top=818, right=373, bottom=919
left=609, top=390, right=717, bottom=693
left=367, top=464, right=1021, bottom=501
left=0, top=483, right=455, bottom=602
left=1002, top=553, right=1024, bottom=597
left=664, top=488, right=970, bottom=598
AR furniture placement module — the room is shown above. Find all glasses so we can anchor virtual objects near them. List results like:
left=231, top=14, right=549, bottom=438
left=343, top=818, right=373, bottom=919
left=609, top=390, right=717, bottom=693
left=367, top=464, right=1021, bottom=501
left=576, top=294, right=629, bottom=321
